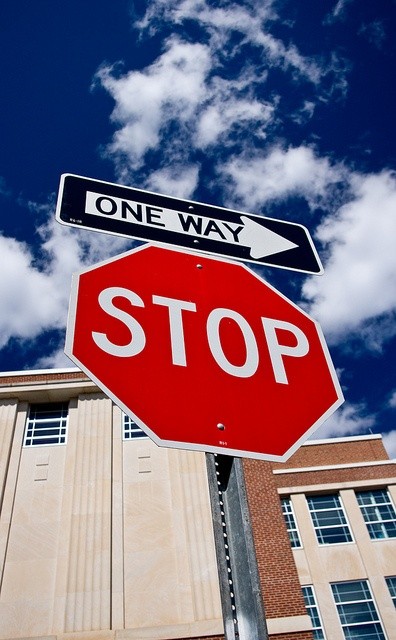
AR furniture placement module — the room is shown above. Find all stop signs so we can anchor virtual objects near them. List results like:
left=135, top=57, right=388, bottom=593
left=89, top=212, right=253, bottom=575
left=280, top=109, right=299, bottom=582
left=63, top=241, right=346, bottom=464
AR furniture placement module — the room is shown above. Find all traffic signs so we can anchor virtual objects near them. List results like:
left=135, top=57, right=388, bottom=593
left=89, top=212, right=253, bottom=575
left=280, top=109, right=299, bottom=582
left=56, top=172, right=325, bottom=279
